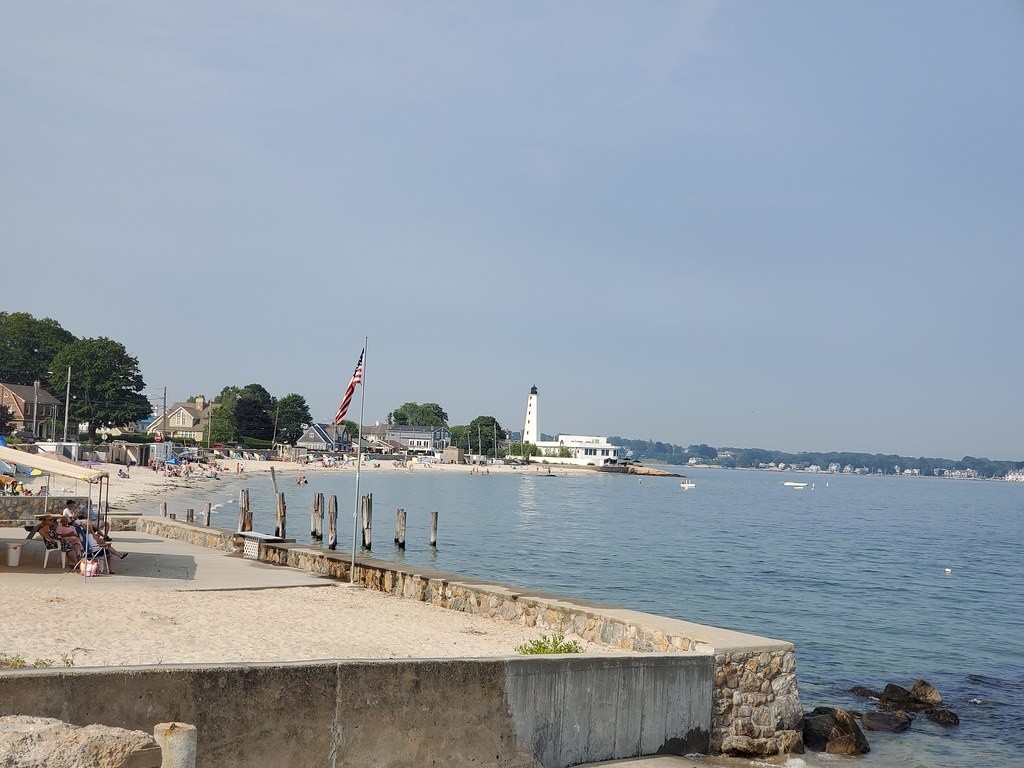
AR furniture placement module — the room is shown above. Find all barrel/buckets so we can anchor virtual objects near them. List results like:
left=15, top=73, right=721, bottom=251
left=6, top=542, right=22, bottom=566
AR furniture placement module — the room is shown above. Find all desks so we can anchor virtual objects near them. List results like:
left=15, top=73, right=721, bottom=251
left=23, top=513, right=63, bottom=540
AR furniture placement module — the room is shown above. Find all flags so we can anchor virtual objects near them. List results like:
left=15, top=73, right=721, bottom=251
left=333, top=345, right=364, bottom=425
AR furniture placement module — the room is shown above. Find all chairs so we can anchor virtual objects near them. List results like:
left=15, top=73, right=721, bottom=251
left=44, top=532, right=72, bottom=569
left=76, top=527, right=112, bottom=572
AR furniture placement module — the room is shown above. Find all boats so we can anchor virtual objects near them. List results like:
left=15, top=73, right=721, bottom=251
left=784, top=481, right=809, bottom=487
left=681, top=483, right=696, bottom=488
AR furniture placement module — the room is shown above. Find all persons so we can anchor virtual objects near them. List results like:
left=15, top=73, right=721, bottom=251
left=40, top=515, right=82, bottom=569
left=0, top=450, right=577, bottom=499
left=82, top=520, right=128, bottom=575
left=62, top=499, right=79, bottom=525
left=80, top=498, right=112, bottom=542
left=56, top=516, right=83, bottom=556
